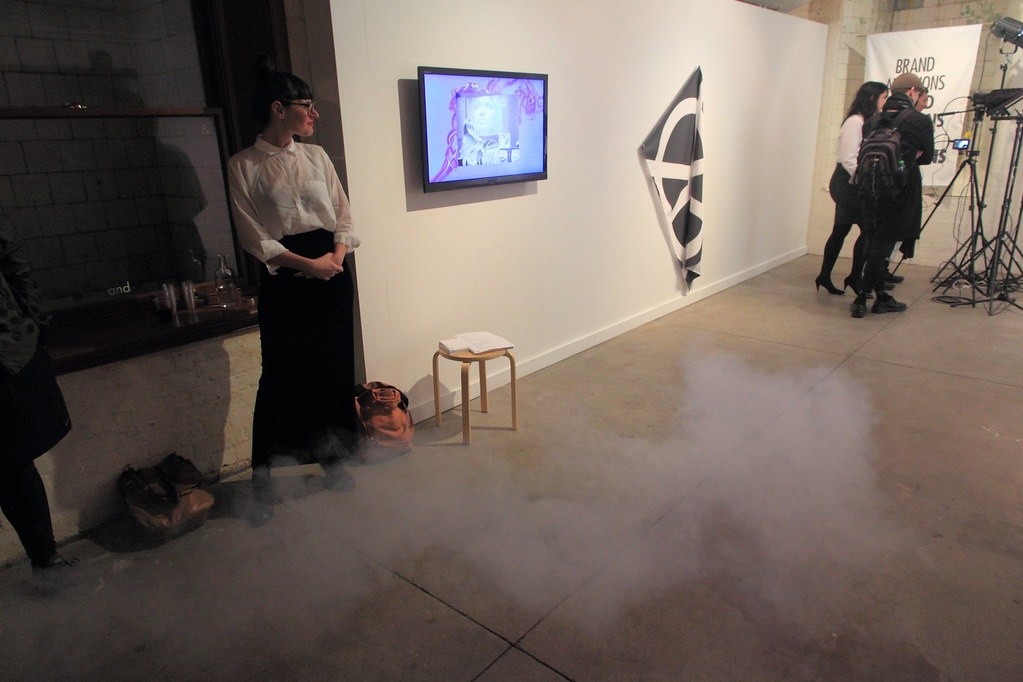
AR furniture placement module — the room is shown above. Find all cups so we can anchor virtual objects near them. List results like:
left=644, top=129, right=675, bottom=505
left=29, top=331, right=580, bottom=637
left=456, top=93, right=522, bottom=166
left=152, top=297, right=168, bottom=311
left=162, top=283, right=177, bottom=313
left=181, top=279, right=195, bottom=310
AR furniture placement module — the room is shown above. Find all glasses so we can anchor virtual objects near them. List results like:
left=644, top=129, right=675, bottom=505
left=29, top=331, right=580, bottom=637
left=280, top=98, right=316, bottom=116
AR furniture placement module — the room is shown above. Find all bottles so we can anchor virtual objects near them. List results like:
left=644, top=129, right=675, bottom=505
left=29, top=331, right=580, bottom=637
left=214, top=253, right=239, bottom=309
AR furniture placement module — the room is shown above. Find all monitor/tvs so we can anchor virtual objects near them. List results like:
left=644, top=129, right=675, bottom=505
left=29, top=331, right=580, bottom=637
left=416, top=66, right=549, bottom=193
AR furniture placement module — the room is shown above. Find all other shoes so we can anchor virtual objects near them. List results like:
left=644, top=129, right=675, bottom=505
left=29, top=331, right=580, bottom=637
left=884, top=284, right=895, bottom=290
left=871, top=296, right=907, bottom=314
left=32, top=550, right=79, bottom=575
left=850, top=303, right=866, bottom=318
left=252, top=461, right=273, bottom=523
left=888, top=272, right=904, bottom=283
left=318, top=461, right=355, bottom=493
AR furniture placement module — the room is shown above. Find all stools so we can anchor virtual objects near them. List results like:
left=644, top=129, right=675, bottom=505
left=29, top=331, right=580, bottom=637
left=432, top=348, right=518, bottom=445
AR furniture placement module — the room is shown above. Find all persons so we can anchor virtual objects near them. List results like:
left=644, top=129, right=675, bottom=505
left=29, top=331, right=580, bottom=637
left=0, top=268, right=72, bottom=599
left=226, top=64, right=361, bottom=524
left=815, top=72, right=935, bottom=319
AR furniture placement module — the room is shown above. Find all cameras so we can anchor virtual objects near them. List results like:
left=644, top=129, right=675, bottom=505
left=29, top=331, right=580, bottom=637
left=952, top=138, right=970, bottom=150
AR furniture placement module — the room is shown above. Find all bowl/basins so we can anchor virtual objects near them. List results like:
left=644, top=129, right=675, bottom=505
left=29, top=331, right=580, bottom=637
left=47, top=313, right=84, bottom=334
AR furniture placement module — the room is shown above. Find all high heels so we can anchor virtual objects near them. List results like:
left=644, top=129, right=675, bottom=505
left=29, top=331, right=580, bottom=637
left=815, top=275, right=845, bottom=295
left=844, top=276, right=874, bottom=299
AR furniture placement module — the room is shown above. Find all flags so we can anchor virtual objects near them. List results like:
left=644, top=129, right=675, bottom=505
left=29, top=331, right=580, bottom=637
left=640, top=67, right=704, bottom=291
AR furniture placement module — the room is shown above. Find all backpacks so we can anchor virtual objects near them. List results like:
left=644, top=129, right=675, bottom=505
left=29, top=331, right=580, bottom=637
left=848, top=108, right=924, bottom=203
left=0, top=272, right=40, bottom=376
left=355, top=381, right=414, bottom=465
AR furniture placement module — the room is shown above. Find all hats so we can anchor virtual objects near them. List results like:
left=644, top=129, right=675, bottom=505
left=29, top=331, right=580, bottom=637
left=890, top=73, right=923, bottom=91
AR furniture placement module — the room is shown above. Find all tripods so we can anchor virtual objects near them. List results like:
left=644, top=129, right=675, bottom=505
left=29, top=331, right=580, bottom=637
left=886, top=61, right=1023, bottom=315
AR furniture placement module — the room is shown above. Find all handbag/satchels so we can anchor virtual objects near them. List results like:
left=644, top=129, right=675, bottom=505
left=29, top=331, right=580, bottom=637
left=119, top=452, right=216, bottom=541
